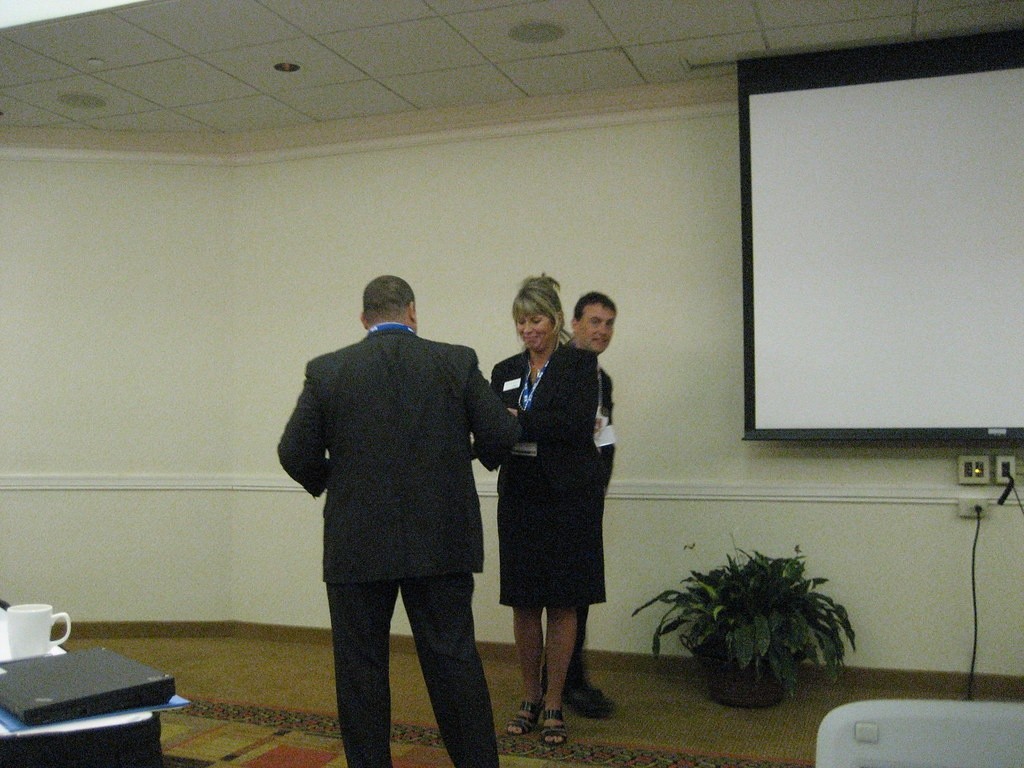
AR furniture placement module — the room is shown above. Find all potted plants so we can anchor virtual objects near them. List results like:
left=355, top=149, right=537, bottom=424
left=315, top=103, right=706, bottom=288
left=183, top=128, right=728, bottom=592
left=631, top=531, right=857, bottom=710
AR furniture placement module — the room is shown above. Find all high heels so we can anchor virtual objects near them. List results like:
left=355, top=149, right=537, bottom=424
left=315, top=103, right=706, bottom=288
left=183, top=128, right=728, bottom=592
left=506, top=699, right=545, bottom=736
left=541, top=708, right=568, bottom=746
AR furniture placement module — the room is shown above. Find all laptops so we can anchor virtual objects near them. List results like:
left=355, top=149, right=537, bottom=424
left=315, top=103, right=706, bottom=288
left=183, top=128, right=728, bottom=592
left=0, top=648, right=177, bottom=726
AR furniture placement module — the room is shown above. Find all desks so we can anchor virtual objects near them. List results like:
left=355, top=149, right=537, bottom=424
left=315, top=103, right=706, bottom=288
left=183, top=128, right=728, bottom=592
left=0, top=711, right=163, bottom=768
left=816, top=699, right=1023, bottom=768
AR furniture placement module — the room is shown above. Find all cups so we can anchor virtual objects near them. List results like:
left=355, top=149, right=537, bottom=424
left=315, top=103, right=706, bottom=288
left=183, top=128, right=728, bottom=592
left=7, top=604, right=71, bottom=660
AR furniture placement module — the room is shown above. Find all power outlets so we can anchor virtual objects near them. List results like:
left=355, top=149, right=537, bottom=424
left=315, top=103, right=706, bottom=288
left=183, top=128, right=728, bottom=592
left=958, top=499, right=987, bottom=519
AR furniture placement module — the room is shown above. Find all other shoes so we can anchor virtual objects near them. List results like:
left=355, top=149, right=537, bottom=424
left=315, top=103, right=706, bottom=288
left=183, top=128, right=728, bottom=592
left=541, top=663, right=615, bottom=718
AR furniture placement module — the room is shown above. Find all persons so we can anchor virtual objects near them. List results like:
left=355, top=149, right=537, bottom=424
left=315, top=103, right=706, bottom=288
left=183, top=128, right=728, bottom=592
left=492, top=273, right=606, bottom=747
left=277, top=276, right=524, bottom=768
left=539, top=291, right=617, bottom=720
